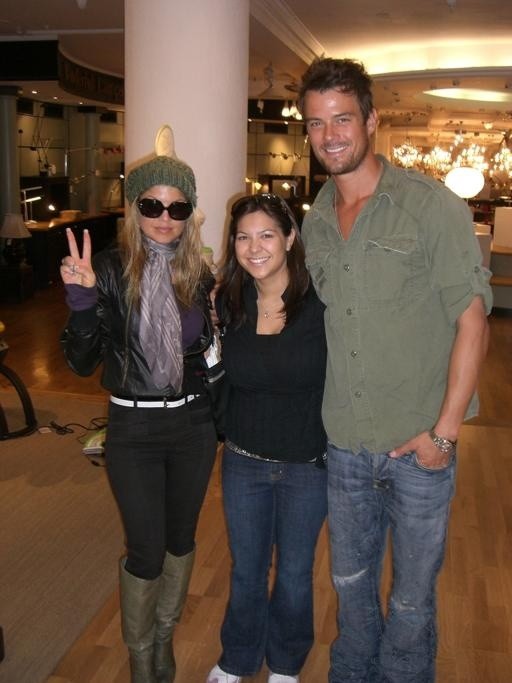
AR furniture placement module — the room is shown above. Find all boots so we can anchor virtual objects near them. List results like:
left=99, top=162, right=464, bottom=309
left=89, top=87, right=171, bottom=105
left=153, top=541, right=196, bottom=683
left=119, top=554, right=163, bottom=682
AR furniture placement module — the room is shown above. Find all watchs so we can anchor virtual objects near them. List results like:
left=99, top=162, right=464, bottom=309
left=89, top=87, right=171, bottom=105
left=428, top=425, right=459, bottom=453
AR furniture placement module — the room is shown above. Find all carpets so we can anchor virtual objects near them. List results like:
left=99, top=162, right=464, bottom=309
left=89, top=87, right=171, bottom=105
left=1, top=384, right=124, bottom=683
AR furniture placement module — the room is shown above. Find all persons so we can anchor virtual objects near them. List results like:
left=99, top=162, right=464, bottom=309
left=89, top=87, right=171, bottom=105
left=210, top=54, right=491, bottom=681
left=58, top=155, right=222, bottom=682
left=206, top=193, right=329, bottom=682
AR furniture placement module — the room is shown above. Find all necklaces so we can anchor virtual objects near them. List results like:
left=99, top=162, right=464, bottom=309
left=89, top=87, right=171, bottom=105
left=257, top=297, right=283, bottom=319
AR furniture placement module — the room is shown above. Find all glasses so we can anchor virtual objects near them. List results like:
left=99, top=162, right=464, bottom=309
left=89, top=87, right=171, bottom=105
left=136, top=197, right=193, bottom=221
left=230, top=192, right=284, bottom=216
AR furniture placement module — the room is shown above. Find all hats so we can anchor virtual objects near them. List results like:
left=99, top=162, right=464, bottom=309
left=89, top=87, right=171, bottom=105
left=125, top=155, right=197, bottom=205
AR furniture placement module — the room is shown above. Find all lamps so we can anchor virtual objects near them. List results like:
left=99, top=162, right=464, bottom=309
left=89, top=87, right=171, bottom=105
left=0, top=212, right=33, bottom=248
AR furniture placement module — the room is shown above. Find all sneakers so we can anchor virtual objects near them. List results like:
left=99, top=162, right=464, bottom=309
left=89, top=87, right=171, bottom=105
left=268, top=671, right=300, bottom=682
left=204, top=664, right=243, bottom=683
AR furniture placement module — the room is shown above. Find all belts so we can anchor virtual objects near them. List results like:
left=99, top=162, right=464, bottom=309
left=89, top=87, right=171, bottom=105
left=106, top=394, right=202, bottom=412
left=224, top=438, right=326, bottom=463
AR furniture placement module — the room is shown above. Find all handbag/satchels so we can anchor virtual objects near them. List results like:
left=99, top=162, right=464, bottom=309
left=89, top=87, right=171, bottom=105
left=205, top=360, right=226, bottom=443
